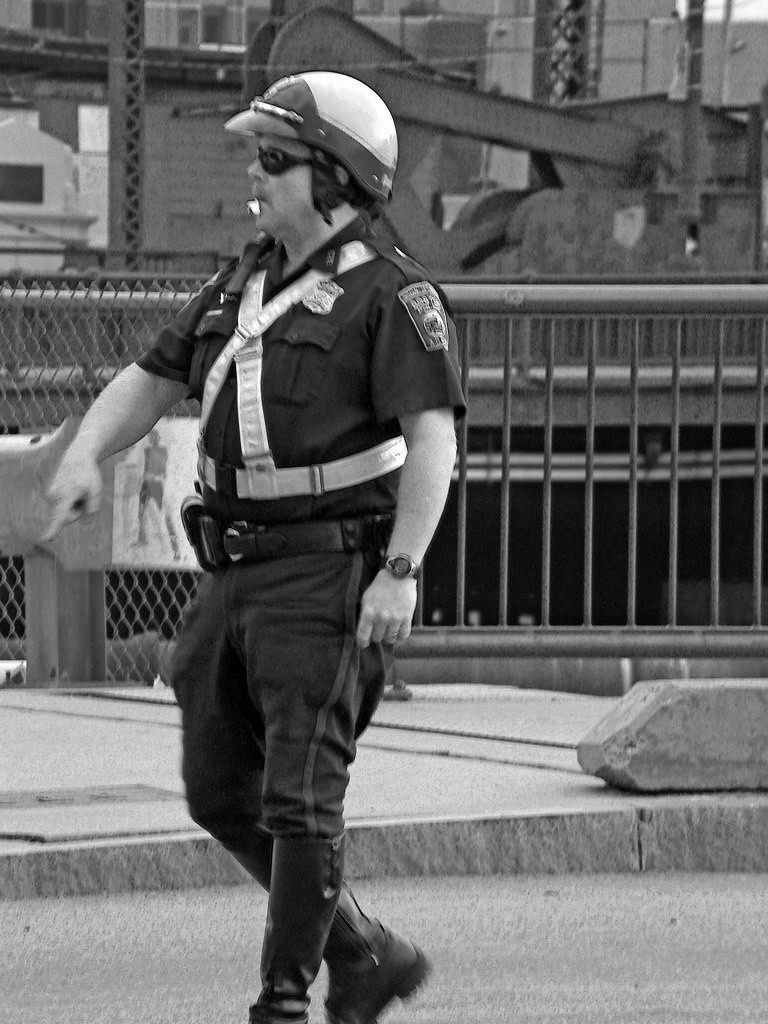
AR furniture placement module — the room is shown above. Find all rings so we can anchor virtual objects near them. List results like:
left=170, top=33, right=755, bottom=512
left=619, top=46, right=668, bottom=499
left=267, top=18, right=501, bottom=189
left=389, top=632, right=399, bottom=639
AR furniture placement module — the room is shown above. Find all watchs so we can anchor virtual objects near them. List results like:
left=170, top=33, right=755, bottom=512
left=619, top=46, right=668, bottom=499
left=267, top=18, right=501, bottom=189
left=380, top=552, right=422, bottom=580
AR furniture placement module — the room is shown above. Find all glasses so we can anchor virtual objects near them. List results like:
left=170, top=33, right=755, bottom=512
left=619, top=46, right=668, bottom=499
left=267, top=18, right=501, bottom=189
left=252, top=144, right=325, bottom=176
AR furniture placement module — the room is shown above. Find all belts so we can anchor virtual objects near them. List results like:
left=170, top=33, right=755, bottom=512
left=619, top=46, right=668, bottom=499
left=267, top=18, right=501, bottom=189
left=218, top=509, right=401, bottom=566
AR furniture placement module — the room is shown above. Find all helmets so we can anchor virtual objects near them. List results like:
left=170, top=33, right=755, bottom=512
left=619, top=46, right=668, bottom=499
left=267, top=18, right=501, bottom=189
left=223, top=70, right=398, bottom=203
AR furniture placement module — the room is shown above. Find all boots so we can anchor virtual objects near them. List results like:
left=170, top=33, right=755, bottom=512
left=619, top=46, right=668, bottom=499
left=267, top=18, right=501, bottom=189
left=221, top=823, right=425, bottom=1024
left=247, top=833, right=347, bottom=1024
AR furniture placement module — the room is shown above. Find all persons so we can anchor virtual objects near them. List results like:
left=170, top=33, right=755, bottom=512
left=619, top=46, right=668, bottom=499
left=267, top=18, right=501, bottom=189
left=40, top=71, right=470, bottom=1024
